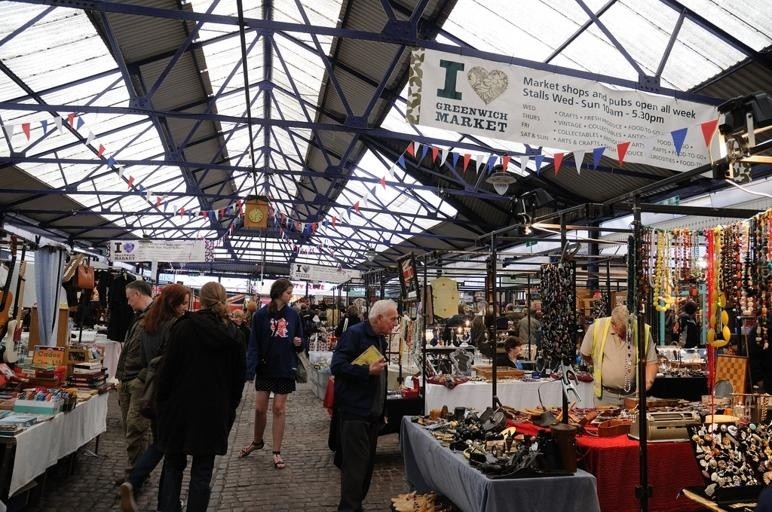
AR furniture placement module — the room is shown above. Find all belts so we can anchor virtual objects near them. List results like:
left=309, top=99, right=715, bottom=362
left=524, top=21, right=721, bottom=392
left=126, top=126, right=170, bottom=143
left=602, top=385, right=636, bottom=395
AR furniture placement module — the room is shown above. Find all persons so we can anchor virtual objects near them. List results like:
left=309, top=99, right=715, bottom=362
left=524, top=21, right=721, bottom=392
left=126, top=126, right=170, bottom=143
left=579, top=304, right=660, bottom=410
left=237, top=276, right=308, bottom=471
left=118, top=283, right=193, bottom=512
left=113, top=278, right=155, bottom=487
left=664, top=298, right=772, bottom=394
left=227, top=293, right=541, bottom=371
left=328, top=298, right=400, bottom=511
left=151, top=281, right=250, bottom=512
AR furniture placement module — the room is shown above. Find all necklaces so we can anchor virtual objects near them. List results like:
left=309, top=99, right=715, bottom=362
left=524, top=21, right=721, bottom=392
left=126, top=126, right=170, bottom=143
left=622, top=314, right=634, bottom=393
left=620, top=206, right=772, bottom=353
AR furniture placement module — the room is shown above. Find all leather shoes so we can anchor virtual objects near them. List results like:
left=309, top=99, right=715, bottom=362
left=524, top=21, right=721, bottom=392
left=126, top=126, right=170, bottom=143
left=390, top=491, right=438, bottom=512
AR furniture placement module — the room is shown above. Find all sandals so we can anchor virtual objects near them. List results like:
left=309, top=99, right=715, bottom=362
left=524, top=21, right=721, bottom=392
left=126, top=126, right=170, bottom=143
left=238, top=440, right=264, bottom=458
left=272, top=451, right=285, bottom=469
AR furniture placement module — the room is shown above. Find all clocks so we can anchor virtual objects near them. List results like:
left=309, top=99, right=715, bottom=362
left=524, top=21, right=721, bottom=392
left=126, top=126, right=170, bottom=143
left=242, top=194, right=270, bottom=229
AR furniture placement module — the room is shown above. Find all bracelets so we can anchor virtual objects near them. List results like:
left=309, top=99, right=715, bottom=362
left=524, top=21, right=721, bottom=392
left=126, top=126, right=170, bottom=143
left=729, top=394, right=771, bottom=425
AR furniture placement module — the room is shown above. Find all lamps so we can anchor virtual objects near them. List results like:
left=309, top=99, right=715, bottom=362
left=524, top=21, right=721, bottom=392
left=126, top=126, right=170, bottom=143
left=485, top=155, right=519, bottom=197
left=516, top=187, right=556, bottom=215
left=712, top=88, right=772, bottom=162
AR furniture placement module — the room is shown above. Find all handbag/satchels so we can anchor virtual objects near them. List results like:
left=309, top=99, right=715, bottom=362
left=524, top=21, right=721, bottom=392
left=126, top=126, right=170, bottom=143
left=74, top=259, right=95, bottom=289
left=138, top=370, right=159, bottom=419
left=296, top=349, right=310, bottom=383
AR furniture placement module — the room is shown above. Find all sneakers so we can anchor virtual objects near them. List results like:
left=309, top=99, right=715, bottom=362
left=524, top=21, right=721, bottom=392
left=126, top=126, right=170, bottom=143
left=120, top=482, right=137, bottom=512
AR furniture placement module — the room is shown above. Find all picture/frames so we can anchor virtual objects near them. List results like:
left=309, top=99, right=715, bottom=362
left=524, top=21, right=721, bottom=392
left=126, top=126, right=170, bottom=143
left=395, top=250, right=421, bottom=304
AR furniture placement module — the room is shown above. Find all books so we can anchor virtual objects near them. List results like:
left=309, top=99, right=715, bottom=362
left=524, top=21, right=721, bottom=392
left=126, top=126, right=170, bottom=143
left=0, top=359, right=116, bottom=439
